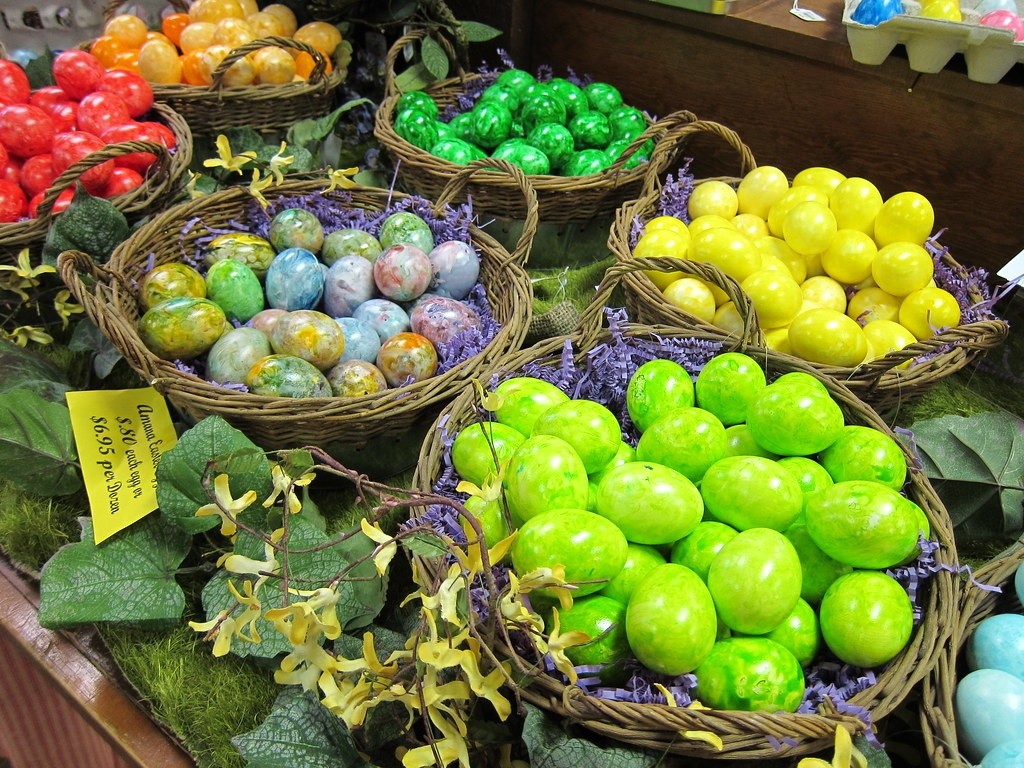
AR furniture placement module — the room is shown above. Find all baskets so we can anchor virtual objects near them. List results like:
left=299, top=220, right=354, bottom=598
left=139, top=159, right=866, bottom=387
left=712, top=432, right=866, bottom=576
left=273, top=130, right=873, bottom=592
left=904, top=522, right=1024, bottom=767
left=404, top=262, right=958, bottom=768
left=77, top=0, right=347, bottom=151
left=56, top=157, right=536, bottom=491
left=605, top=122, right=1009, bottom=423
left=373, top=27, right=697, bottom=254
left=0, top=100, right=184, bottom=328
